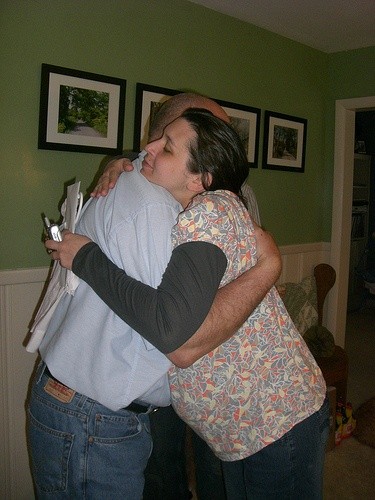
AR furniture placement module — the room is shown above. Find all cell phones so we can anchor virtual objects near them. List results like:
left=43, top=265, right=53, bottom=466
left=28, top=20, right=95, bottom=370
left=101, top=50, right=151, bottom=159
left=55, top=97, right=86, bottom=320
left=45, top=218, right=63, bottom=243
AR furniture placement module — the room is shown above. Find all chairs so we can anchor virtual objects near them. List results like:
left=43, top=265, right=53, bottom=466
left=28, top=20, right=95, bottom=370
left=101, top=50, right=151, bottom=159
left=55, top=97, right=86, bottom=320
left=276, top=275, right=348, bottom=451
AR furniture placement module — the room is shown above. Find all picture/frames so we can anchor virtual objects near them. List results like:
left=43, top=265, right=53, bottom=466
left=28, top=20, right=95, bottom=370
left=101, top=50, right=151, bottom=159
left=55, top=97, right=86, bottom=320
left=37, top=63, right=127, bottom=156
left=262, top=109, right=307, bottom=173
left=134, top=82, right=184, bottom=155
left=209, top=97, right=261, bottom=169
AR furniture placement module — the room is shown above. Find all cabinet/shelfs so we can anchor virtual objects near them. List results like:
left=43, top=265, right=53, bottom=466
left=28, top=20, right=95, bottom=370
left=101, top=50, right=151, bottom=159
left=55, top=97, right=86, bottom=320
left=347, top=153, right=371, bottom=311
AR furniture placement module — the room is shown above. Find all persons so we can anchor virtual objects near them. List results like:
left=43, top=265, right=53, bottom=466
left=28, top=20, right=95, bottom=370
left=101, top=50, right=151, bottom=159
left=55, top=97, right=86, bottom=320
left=41, top=108, right=331, bottom=500
left=22, top=90, right=281, bottom=500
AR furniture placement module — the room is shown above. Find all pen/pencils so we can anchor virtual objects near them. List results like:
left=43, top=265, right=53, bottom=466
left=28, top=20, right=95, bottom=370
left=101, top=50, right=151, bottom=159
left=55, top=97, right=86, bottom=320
left=44, top=216, right=51, bottom=228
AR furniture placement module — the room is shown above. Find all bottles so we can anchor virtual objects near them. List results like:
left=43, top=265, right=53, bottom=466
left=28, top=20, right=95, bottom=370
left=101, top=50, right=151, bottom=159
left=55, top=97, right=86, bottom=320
left=336, top=402, right=352, bottom=428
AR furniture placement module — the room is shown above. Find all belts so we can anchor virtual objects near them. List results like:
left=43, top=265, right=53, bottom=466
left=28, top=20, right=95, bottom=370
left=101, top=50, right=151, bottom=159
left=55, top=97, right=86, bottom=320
left=44, top=364, right=158, bottom=417
